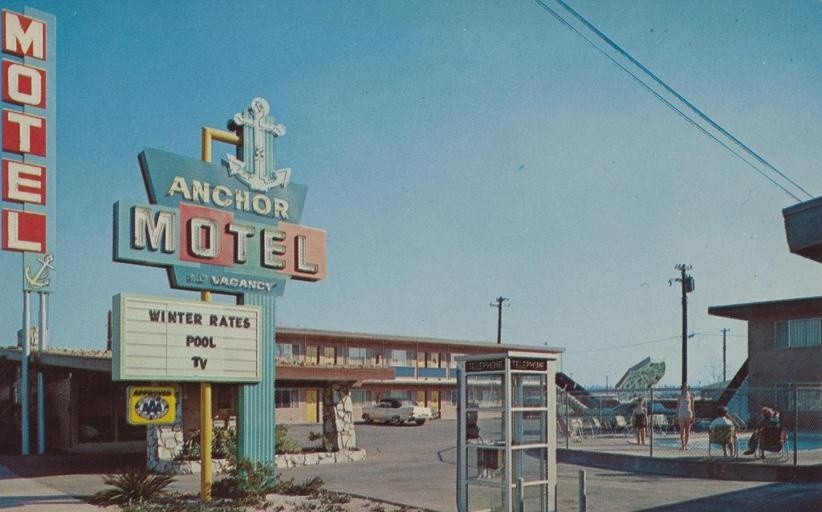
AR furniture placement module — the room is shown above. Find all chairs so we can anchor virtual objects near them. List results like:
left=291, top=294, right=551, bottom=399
left=755, top=422, right=789, bottom=464
left=559, top=414, right=629, bottom=442
left=709, top=425, right=739, bottom=461
left=648, top=414, right=672, bottom=435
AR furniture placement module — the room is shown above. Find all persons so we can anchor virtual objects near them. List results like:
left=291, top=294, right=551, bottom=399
left=742, top=406, right=781, bottom=455
left=708, top=406, right=741, bottom=457
left=675, top=383, right=696, bottom=450
left=631, top=397, right=648, bottom=446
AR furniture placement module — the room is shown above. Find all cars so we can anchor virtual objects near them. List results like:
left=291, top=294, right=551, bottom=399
left=362, top=399, right=431, bottom=426
